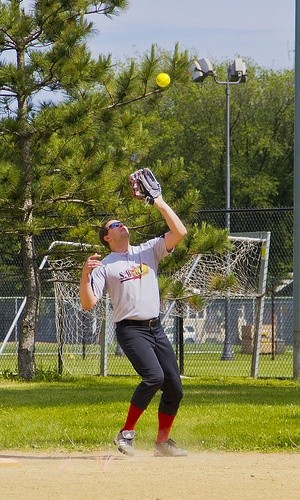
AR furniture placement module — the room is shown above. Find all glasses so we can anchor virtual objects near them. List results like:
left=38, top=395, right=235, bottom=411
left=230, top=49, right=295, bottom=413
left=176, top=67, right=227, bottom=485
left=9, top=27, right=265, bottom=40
left=104, top=222, right=123, bottom=236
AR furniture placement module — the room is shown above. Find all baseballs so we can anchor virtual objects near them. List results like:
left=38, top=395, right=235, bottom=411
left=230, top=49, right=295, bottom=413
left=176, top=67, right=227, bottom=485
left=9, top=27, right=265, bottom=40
left=156, top=72, right=170, bottom=88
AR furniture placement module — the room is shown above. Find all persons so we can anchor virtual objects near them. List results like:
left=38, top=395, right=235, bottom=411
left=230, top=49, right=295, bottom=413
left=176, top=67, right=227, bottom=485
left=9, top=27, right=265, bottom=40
left=80, top=169, right=188, bottom=460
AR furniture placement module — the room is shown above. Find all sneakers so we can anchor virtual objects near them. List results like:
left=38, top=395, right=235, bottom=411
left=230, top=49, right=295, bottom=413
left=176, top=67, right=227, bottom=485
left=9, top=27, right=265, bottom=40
left=153, top=438, right=189, bottom=457
left=113, top=430, right=136, bottom=456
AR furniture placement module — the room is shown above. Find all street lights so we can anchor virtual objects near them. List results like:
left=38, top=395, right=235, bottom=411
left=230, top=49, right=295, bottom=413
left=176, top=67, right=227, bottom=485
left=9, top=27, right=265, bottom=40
left=187, top=58, right=249, bottom=359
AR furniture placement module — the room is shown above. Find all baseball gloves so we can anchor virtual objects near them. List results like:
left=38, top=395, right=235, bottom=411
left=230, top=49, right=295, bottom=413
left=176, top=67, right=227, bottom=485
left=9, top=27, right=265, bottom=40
left=129, top=168, right=162, bottom=206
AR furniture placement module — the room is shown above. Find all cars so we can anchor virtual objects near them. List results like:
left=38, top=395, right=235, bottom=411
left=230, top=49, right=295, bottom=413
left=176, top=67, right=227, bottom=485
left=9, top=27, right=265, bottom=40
left=162, top=325, right=197, bottom=344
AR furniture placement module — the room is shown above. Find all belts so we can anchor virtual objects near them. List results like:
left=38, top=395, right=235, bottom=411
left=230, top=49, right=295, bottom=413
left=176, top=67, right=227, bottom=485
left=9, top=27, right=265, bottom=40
left=116, top=316, right=160, bottom=327
